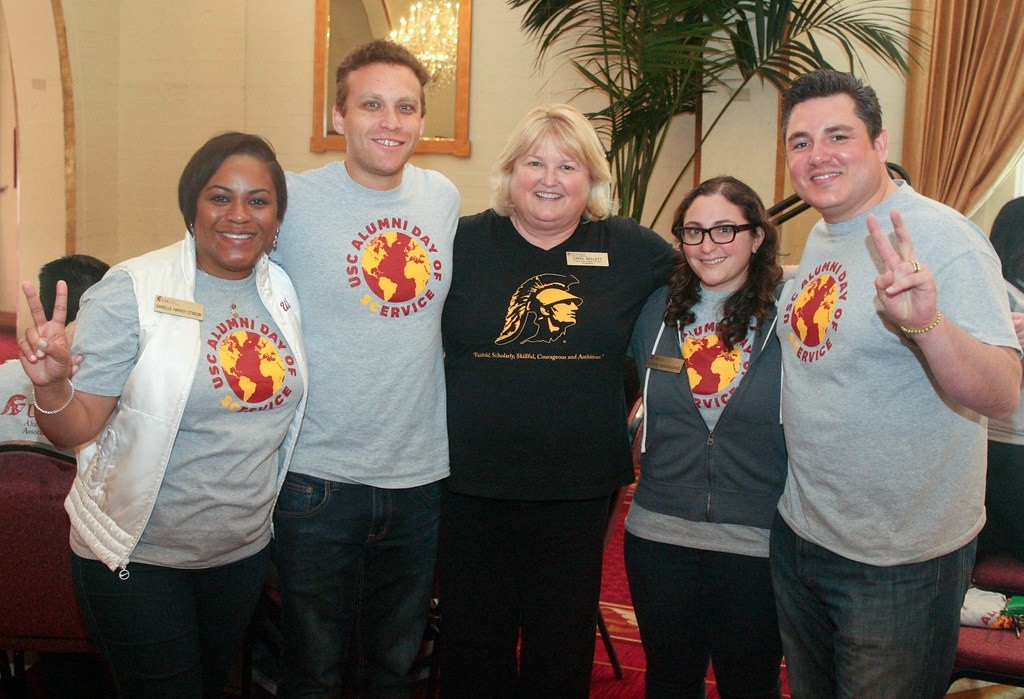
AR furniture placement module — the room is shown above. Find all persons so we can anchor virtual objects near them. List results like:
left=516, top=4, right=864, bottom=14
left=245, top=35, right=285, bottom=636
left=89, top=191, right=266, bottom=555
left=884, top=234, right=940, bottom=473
left=769, top=68, right=1024, bottom=699
left=420, top=104, right=801, bottom=699
left=70, top=42, right=461, bottom=699
left=14, top=131, right=311, bottom=699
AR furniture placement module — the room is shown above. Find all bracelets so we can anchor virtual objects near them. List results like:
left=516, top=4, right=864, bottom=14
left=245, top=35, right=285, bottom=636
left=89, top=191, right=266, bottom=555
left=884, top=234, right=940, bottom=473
left=899, top=306, right=941, bottom=334
left=32, top=378, right=75, bottom=414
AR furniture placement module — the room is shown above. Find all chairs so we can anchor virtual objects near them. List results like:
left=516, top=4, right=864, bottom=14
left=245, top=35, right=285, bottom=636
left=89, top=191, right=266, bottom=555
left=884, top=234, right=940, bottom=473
left=0, top=439, right=280, bottom=699
left=942, top=554, right=1024, bottom=699
left=592, top=399, right=644, bottom=680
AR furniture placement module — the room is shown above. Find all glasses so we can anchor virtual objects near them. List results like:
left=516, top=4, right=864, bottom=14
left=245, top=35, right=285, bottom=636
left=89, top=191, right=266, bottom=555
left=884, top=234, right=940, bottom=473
left=672, top=224, right=757, bottom=245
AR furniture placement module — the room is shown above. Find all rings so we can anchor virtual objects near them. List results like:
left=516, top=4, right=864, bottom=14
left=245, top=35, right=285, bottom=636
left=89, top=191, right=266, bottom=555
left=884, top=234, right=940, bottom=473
left=17, top=338, right=25, bottom=346
left=908, top=258, right=920, bottom=272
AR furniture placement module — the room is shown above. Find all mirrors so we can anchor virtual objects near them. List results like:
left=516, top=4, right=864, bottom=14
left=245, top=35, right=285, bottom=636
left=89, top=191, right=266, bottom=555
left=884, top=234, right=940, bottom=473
left=310, top=0, right=473, bottom=159
